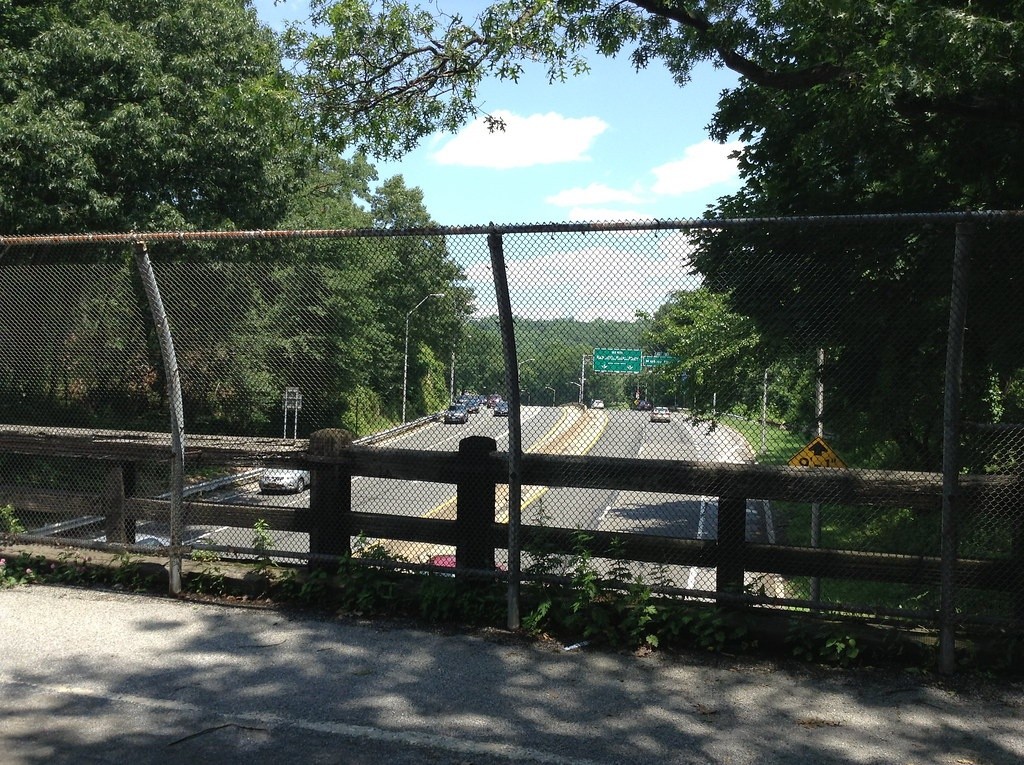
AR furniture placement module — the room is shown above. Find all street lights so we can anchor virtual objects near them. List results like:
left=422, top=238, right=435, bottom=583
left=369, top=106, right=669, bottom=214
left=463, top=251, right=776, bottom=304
left=566, top=381, right=582, bottom=403
left=545, top=387, right=555, bottom=408
left=402, top=294, right=445, bottom=426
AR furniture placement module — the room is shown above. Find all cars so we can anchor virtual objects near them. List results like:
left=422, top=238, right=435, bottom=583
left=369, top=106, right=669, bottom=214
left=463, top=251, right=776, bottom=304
left=93, top=534, right=184, bottom=549
left=456, top=393, right=503, bottom=414
left=495, top=402, right=508, bottom=417
left=443, top=403, right=468, bottom=425
left=637, top=400, right=654, bottom=410
left=650, top=406, right=670, bottom=423
left=591, top=400, right=604, bottom=409
left=259, top=468, right=311, bottom=494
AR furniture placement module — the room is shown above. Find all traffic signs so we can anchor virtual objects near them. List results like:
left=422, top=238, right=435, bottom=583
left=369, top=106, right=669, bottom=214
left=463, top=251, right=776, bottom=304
left=592, top=348, right=643, bottom=373
left=643, top=354, right=680, bottom=367
